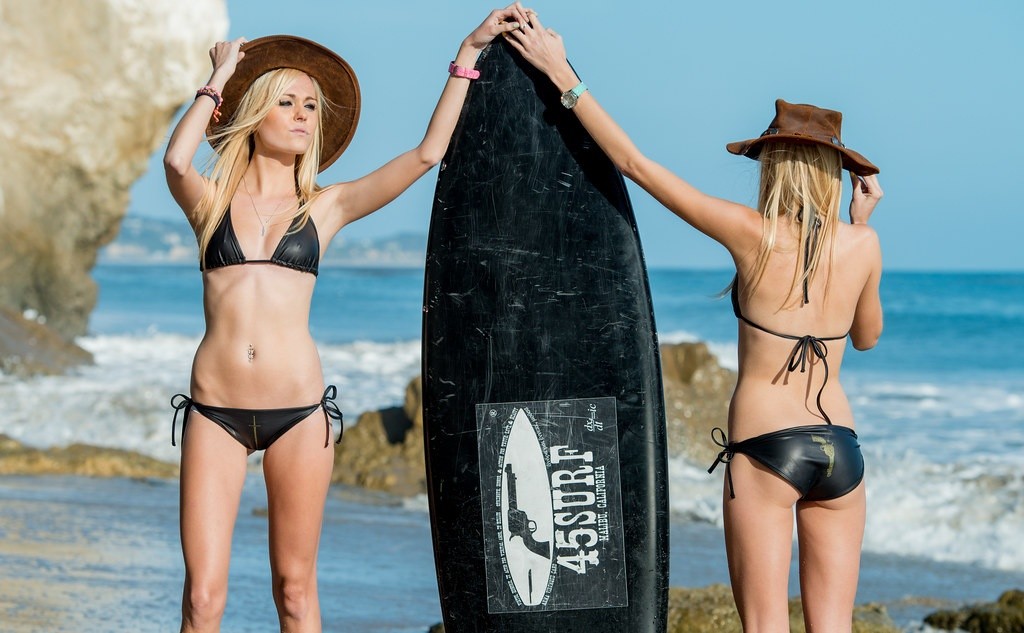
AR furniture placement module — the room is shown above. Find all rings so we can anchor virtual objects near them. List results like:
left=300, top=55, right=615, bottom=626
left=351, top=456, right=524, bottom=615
left=522, top=23, right=528, bottom=30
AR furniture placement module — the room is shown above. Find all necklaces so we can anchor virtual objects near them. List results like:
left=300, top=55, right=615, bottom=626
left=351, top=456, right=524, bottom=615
left=241, top=174, right=300, bottom=237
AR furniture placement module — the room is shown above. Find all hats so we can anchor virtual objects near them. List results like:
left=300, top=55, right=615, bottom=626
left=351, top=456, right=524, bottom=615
left=727, top=98, right=879, bottom=177
left=206, top=34, right=362, bottom=176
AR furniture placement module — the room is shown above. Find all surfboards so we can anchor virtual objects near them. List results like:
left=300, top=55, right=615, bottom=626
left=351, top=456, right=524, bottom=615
left=421, top=23, right=671, bottom=631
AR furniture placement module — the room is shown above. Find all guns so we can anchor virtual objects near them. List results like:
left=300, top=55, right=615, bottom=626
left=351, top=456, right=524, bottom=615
left=504, top=463, right=550, bottom=560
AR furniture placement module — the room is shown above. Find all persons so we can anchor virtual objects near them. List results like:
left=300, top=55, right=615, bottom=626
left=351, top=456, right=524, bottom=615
left=162, top=0, right=539, bottom=633
left=501, top=0, right=884, bottom=633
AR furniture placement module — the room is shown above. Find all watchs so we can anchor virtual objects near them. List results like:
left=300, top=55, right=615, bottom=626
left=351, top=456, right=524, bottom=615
left=561, top=82, right=587, bottom=109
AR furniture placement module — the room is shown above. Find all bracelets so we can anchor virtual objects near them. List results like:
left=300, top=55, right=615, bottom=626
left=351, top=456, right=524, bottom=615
left=194, top=86, right=224, bottom=123
left=448, top=61, right=480, bottom=79
